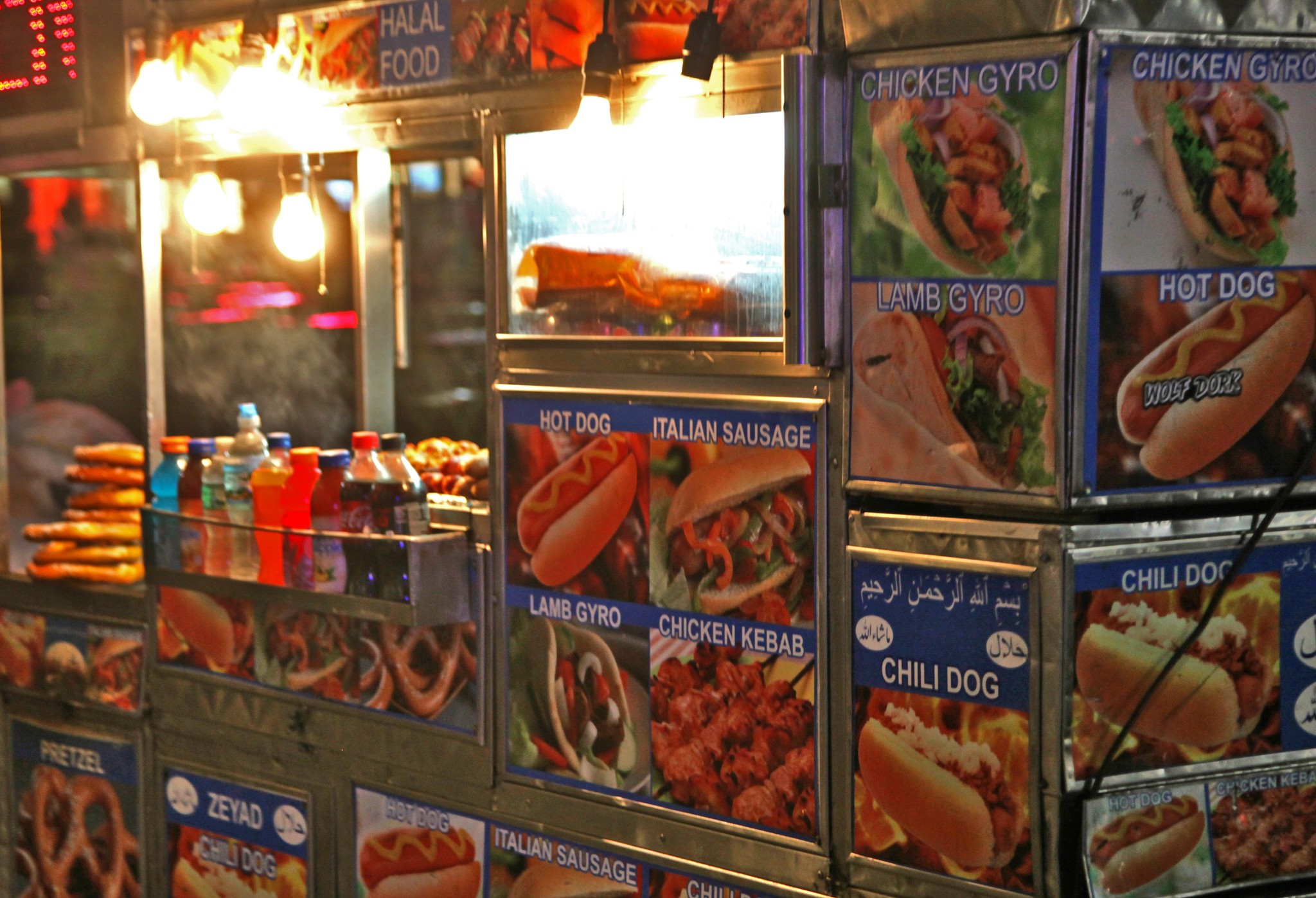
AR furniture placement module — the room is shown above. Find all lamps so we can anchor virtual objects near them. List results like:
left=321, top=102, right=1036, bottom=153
left=271, top=159, right=326, bottom=263
left=125, top=2, right=183, bottom=128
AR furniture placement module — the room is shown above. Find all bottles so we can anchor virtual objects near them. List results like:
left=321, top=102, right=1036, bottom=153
left=150, top=404, right=430, bottom=604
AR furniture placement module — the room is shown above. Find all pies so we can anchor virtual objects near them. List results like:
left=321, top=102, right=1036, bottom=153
left=1, top=410, right=170, bottom=589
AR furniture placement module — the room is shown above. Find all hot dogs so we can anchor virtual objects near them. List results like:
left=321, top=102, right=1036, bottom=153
left=834, top=5, right=1314, bottom=898
left=487, top=384, right=824, bottom=632
